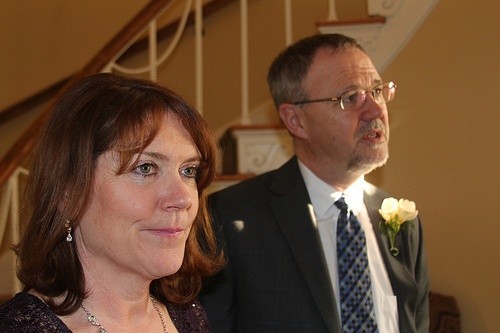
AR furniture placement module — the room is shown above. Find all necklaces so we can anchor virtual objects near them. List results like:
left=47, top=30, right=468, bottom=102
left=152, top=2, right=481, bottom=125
left=80, top=294, right=169, bottom=333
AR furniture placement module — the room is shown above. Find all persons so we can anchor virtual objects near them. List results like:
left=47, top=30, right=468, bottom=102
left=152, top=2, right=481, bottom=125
left=195, top=34, right=431, bottom=333
left=0, top=73, right=228, bottom=333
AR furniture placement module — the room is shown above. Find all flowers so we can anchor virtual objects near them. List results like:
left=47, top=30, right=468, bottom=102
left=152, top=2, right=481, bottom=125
left=378, top=197, right=419, bottom=257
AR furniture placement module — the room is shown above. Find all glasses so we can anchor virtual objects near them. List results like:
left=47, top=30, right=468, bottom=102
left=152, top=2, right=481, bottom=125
left=292, top=81, right=397, bottom=111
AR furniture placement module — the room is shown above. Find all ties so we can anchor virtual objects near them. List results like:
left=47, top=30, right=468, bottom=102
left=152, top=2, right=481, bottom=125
left=334, top=197, right=381, bottom=333
